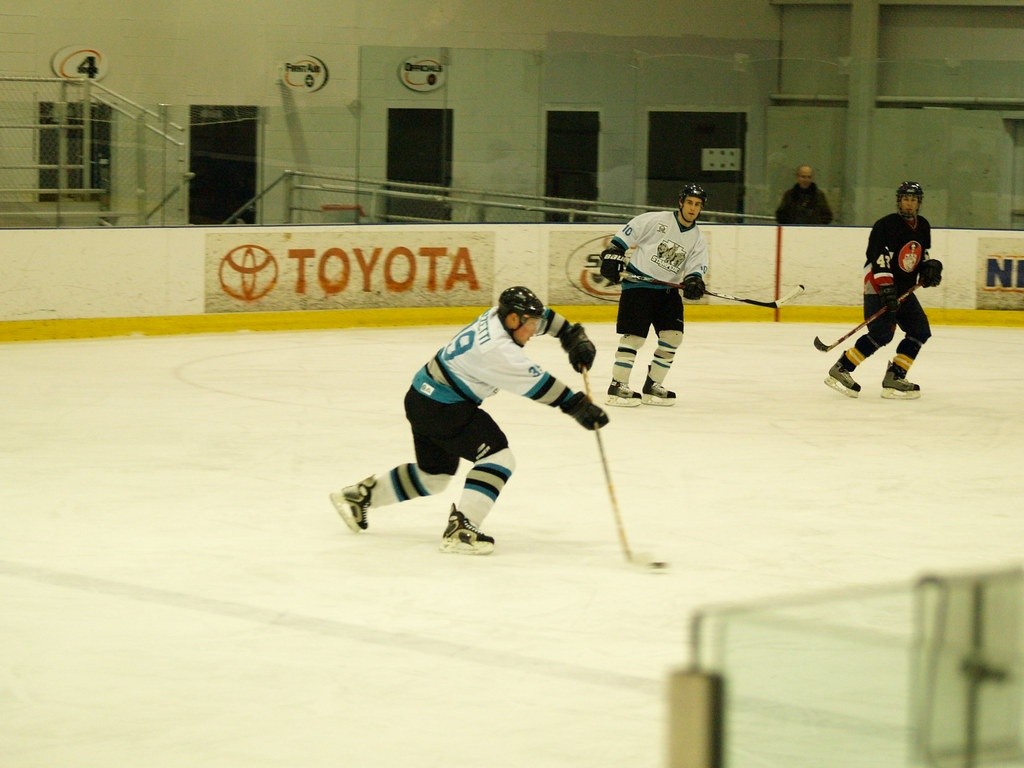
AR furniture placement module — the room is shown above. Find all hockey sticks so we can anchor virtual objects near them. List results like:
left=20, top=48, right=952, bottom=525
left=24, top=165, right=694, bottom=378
left=580, top=366, right=668, bottom=572
left=601, top=263, right=808, bottom=310
left=813, top=282, right=923, bottom=353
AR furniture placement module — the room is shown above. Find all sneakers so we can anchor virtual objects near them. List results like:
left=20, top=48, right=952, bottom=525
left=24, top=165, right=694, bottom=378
left=331, top=474, right=378, bottom=533
left=881, top=361, right=921, bottom=399
left=824, top=351, right=861, bottom=398
left=641, top=365, right=676, bottom=406
left=606, top=378, right=642, bottom=406
left=443, top=503, right=494, bottom=555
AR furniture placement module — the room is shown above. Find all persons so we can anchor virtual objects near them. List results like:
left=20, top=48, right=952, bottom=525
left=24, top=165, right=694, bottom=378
left=825, top=182, right=942, bottom=399
left=600, top=182, right=709, bottom=406
left=775, top=166, right=833, bottom=226
left=330, top=287, right=609, bottom=552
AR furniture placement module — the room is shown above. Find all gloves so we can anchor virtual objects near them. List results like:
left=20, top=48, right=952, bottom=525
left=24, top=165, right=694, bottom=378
left=918, top=258, right=942, bottom=288
left=683, top=272, right=704, bottom=300
left=561, top=323, right=596, bottom=374
left=878, top=285, right=901, bottom=312
left=561, top=391, right=610, bottom=430
left=600, top=249, right=625, bottom=284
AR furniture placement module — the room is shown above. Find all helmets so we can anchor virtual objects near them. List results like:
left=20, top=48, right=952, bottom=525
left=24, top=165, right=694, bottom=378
left=499, top=286, right=544, bottom=325
left=679, top=183, right=709, bottom=209
left=897, top=182, right=923, bottom=203
